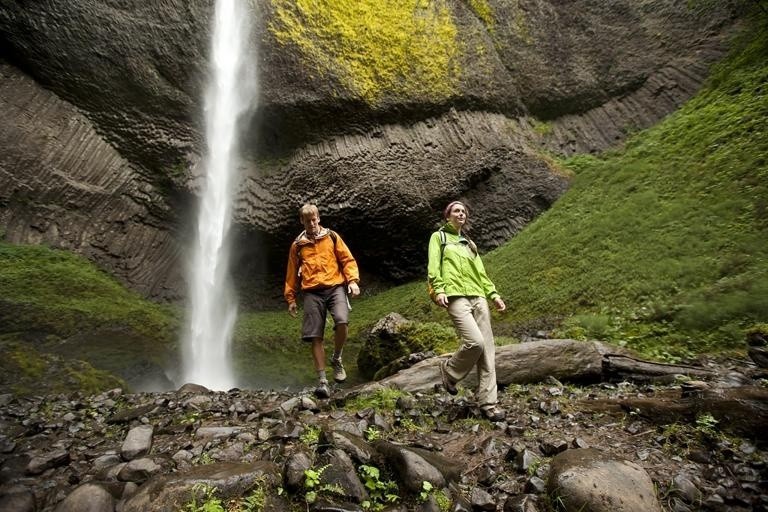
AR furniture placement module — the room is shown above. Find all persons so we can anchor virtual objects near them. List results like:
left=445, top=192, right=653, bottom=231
left=427, top=202, right=506, bottom=420
left=284, top=204, right=360, bottom=398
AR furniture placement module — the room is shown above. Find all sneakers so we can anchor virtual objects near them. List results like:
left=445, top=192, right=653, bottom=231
left=440, top=360, right=458, bottom=395
left=313, top=379, right=331, bottom=400
left=480, top=406, right=506, bottom=421
left=329, top=353, right=348, bottom=383
